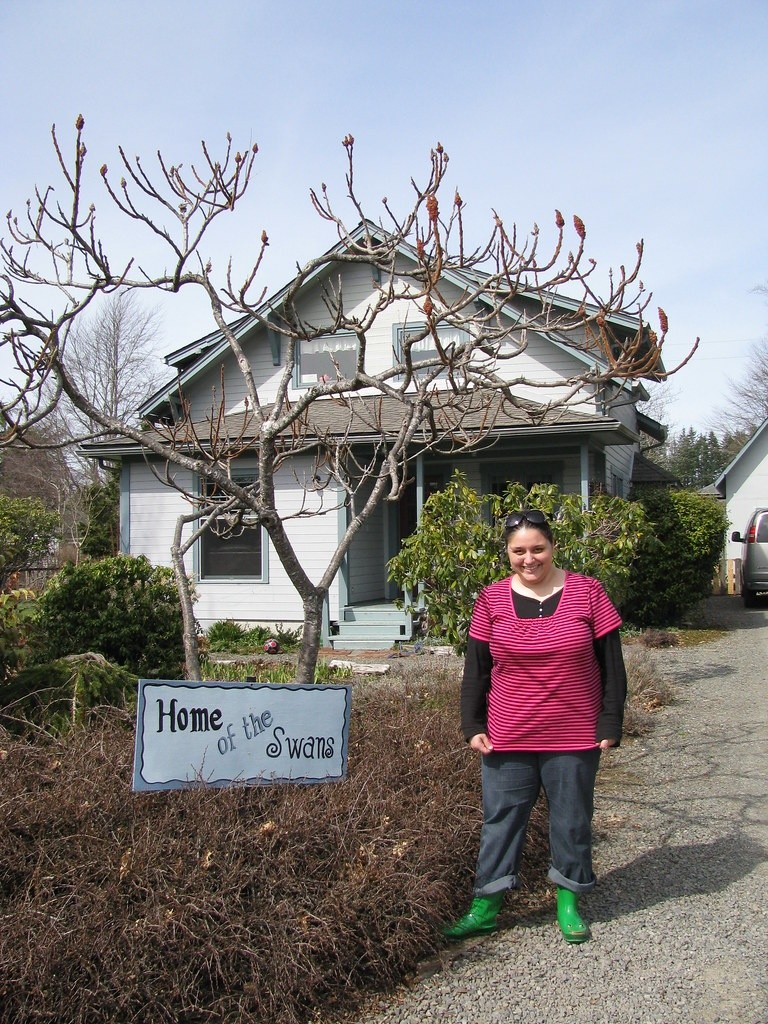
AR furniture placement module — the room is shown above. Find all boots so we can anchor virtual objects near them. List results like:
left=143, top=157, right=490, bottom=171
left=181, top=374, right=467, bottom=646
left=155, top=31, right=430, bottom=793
left=556, top=884, right=588, bottom=943
left=438, top=889, right=504, bottom=940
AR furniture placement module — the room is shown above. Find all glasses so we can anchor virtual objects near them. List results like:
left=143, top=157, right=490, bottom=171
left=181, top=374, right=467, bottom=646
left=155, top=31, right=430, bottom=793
left=505, top=510, right=549, bottom=529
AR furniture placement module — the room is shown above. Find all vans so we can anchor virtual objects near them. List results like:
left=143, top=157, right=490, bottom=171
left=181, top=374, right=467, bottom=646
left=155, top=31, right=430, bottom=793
left=731, top=509, right=768, bottom=609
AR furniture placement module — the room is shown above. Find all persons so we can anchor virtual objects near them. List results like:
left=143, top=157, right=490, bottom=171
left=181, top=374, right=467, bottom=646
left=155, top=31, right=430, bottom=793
left=439, top=511, right=627, bottom=943
